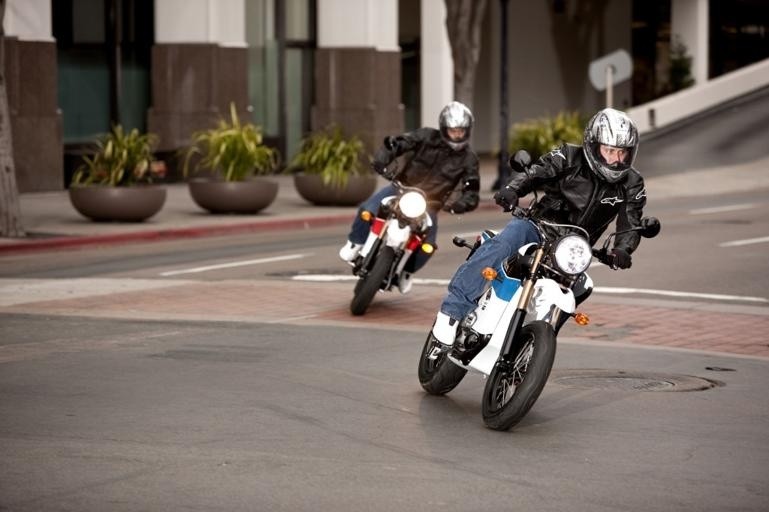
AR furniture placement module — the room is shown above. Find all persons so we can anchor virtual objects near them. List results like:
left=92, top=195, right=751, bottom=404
left=337, top=101, right=481, bottom=295
left=432, top=108, right=648, bottom=352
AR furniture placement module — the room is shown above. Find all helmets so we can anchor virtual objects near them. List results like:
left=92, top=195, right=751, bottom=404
left=438, top=101, right=475, bottom=152
left=582, top=107, right=639, bottom=183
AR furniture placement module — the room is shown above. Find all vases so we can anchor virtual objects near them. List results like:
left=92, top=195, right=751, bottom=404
left=70, top=184, right=165, bottom=221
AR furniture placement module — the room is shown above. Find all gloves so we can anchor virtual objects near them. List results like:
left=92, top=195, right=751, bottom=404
left=451, top=201, right=466, bottom=214
left=496, top=183, right=517, bottom=213
left=611, top=247, right=633, bottom=270
left=373, top=158, right=385, bottom=172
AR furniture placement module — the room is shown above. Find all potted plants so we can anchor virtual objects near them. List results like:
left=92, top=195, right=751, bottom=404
left=178, top=98, right=377, bottom=216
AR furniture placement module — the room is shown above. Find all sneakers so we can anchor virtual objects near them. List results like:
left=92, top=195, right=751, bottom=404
left=432, top=311, right=460, bottom=345
left=339, top=240, right=363, bottom=262
left=398, top=270, right=413, bottom=294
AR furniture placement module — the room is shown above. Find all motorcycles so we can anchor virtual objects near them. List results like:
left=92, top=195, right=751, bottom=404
left=341, top=134, right=480, bottom=314
left=418, top=150, right=662, bottom=427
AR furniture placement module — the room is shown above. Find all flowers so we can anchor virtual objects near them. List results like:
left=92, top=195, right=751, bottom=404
left=70, top=121, right=169, bottom=188
